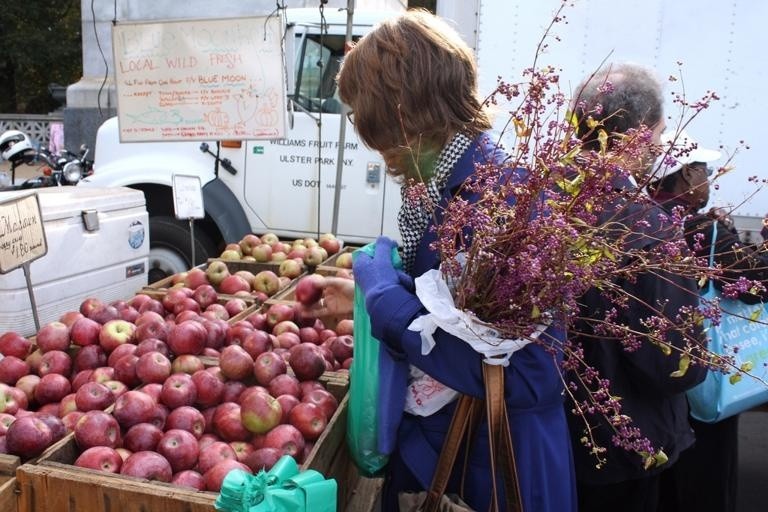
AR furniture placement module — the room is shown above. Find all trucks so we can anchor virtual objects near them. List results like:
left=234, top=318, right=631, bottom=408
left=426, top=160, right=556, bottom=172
left=76, top=2, right=763, bottom=288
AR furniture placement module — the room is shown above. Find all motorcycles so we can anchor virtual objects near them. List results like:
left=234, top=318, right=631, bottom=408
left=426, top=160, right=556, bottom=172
left=1, top=129, right=92, bottom=188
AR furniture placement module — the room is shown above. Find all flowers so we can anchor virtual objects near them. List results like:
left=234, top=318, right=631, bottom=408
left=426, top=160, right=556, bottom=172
left=394, top=0, right=766, bottom=471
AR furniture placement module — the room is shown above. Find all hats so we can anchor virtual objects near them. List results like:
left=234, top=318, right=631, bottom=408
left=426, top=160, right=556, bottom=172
left=645, top=130, right=721, bottom=182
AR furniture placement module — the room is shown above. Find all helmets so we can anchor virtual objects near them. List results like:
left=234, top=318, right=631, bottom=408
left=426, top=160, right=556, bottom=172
left=0, top=130, right=33, bottom=160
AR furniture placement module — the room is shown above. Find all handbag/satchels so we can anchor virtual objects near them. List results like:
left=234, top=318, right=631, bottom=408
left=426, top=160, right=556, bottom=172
left=399, top=357, right=521, bottom=511
left=681, top=215, right=767, bottom=423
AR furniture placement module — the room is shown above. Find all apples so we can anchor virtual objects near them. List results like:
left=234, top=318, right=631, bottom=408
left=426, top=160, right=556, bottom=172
left=0, top=233, right=354, bottom=487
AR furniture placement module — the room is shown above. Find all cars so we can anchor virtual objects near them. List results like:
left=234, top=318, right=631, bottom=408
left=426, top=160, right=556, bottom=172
left=0, top=185, right=151, bottom=337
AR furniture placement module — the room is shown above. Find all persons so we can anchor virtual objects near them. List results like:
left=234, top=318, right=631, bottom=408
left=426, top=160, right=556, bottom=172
left=335, top=7, right=579, bottom=512
left=547, top=66, right=709, bottom=512
left=647, top=133, right=768, bottom=512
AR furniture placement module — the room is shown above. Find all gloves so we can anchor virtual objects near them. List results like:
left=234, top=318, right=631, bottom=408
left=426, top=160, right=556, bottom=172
left=352, top=238, right=414, bottom=314
